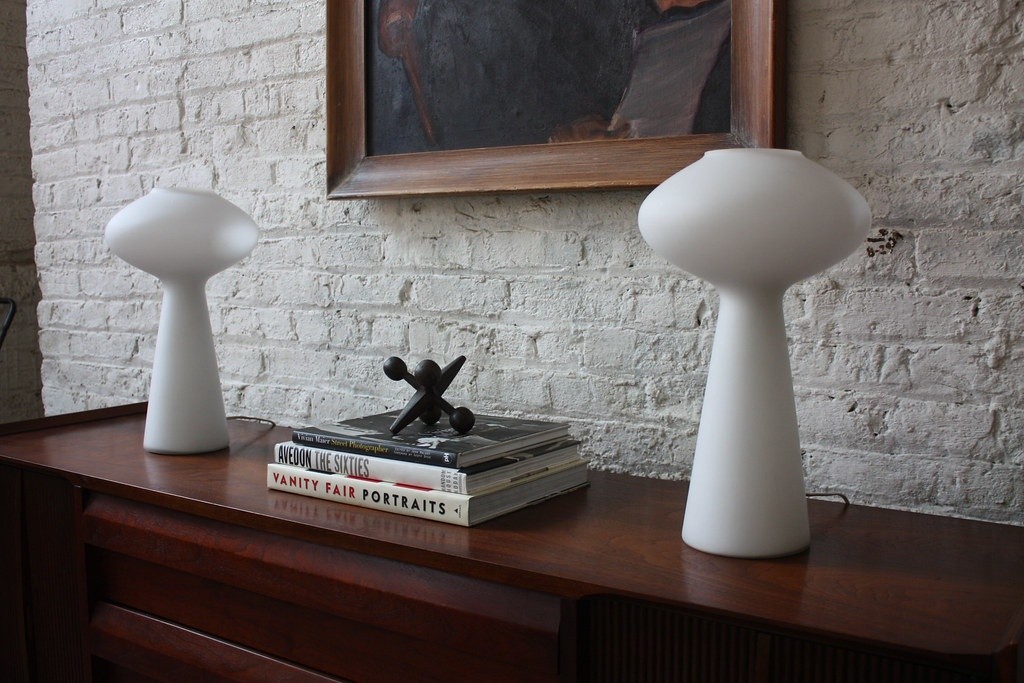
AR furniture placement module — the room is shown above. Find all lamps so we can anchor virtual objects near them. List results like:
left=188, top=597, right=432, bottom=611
left=104, top=184, right=260, bottom=453
left=637, top=147, right=871, bottom=557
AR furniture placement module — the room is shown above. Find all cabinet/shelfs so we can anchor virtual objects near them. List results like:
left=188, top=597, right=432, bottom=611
left=0, top=401, right=1024, bottom=682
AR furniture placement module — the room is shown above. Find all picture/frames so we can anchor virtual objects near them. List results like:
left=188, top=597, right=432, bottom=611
left=326, top=0, right=786, bottom=201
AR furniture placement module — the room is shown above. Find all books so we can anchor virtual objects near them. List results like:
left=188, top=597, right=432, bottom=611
left=266, top=407, right=590, bottom=526
